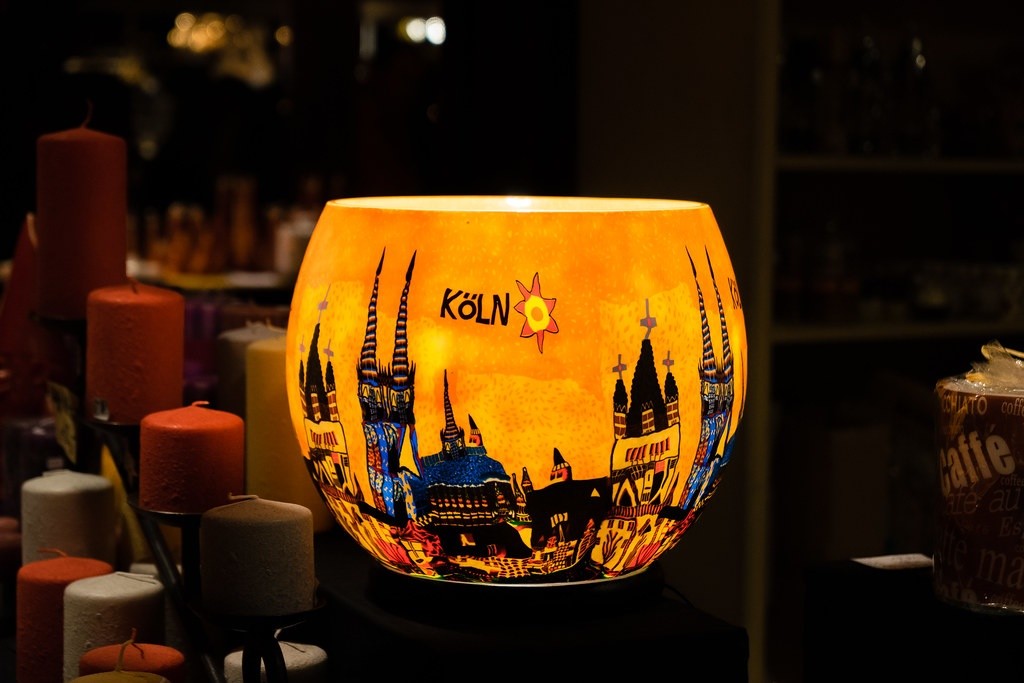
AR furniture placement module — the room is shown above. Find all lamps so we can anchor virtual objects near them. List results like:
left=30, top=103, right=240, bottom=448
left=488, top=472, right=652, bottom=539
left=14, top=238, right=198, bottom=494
left=291, top=197, right=749, bottom=593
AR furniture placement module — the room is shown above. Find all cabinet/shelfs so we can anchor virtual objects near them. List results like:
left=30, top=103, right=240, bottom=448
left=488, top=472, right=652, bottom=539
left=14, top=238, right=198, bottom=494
left=566, top=0, right=1024, bottom=679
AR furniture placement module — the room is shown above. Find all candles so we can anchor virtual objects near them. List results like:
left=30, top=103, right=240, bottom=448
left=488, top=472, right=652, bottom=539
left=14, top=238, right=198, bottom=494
left=18, top=104, right=330, bottom=683
